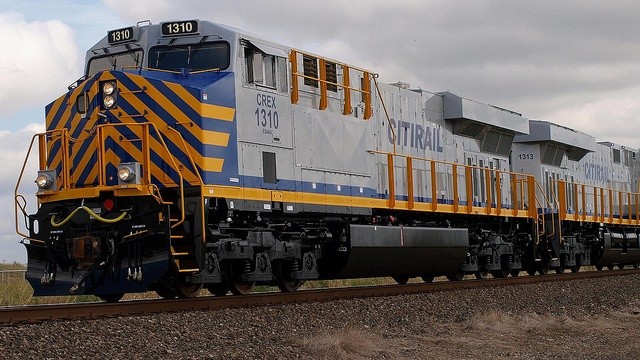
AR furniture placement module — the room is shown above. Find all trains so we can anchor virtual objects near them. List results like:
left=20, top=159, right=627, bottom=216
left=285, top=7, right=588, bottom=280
left=13, top=19, right=639, bottom=301
